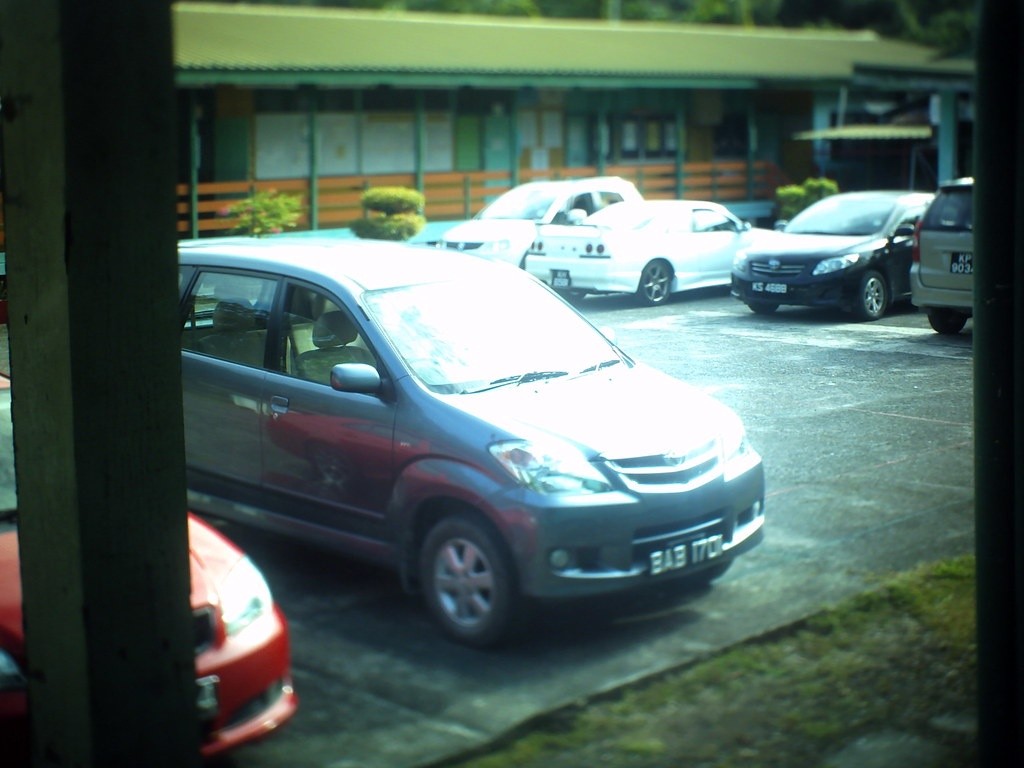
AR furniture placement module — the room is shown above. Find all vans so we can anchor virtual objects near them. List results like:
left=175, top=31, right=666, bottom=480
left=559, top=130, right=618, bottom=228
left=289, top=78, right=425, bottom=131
left=170, top=237, right=769, bottom=649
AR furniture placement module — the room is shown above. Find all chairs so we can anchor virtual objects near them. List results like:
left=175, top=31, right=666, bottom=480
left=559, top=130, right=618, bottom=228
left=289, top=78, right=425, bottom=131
left=198, top=292, right=378, bottom=388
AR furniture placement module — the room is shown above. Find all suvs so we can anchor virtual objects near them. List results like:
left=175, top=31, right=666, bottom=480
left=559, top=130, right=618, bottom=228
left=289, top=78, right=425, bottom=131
left=434, top=177, right=644, bottom=269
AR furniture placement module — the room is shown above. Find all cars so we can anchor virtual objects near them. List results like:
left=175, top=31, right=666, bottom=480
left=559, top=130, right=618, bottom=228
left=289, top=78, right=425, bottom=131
left=1, top=374, right=300, bottom=766
left=728, top=189, right=938, bottom=325
left=905, top=175, right=977, bottom=335
left=520, top=199, right=775, bottom=305
